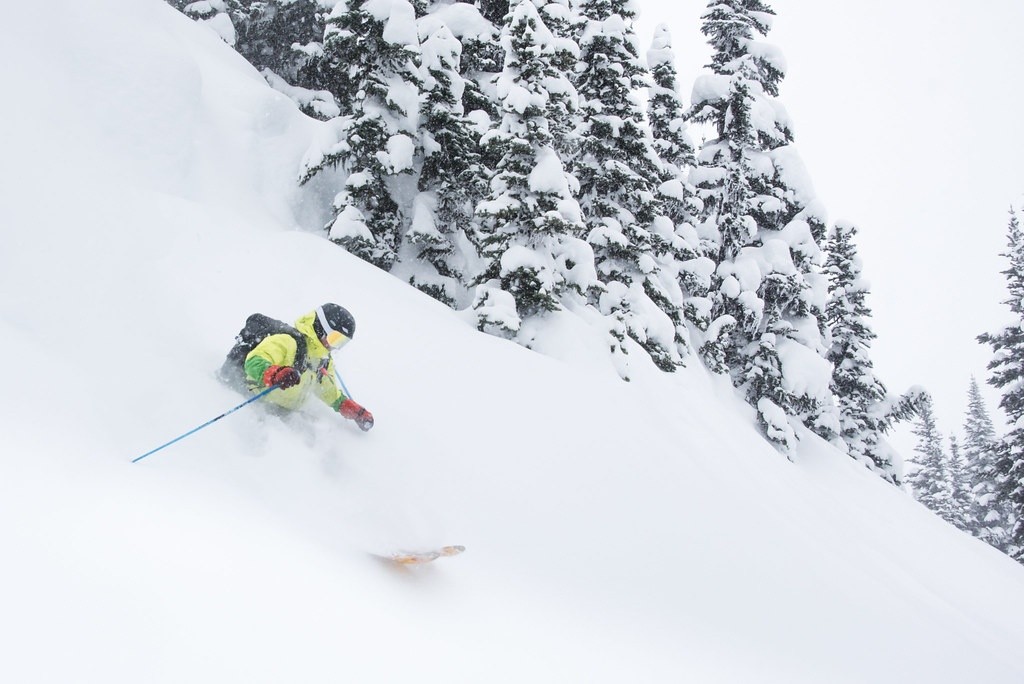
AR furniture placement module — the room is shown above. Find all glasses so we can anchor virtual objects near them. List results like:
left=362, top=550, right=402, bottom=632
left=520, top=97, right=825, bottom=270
left=315, top=305, right=351, bottom=350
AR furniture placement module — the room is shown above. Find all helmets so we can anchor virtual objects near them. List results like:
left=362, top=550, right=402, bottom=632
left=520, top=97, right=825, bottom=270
left=312, top=303, right=356, bottom=351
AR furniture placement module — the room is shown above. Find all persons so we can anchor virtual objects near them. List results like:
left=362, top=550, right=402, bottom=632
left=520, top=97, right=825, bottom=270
left=244, top=302, right=375, bottom=433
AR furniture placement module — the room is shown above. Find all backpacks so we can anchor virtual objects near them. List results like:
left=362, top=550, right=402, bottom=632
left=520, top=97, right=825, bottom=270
left=217, top=314, right=330, bottom=399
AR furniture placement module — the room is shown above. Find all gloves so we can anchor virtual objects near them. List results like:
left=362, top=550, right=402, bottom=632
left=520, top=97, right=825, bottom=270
left=263, top=364, right=301, bottom=391
left=339, top=396, right=374, bottom=432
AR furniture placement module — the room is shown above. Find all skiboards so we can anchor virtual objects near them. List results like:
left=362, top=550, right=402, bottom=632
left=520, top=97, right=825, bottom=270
left=358, top=537, right=470, bottom=566
